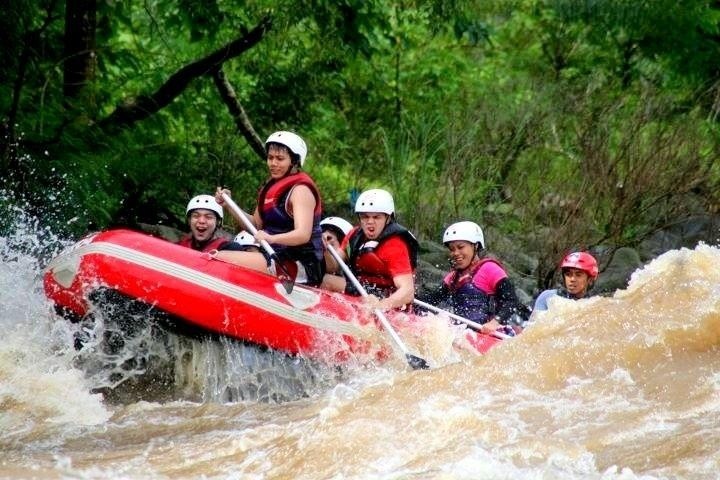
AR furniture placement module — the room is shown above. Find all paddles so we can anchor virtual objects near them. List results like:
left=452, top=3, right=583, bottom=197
left=216, top=189, right=297, bottom=281
left=328, top=241, right=429, bottom=373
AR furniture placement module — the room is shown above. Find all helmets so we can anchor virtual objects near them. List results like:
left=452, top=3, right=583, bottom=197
left=560, top=251, right=598, bottom=282
left=355, top=188, right=396, bottom=218
left=233, top=230, right=262, bottom=248
left=442, top=221, right=485, bottom=251
left=265, top=130, right=308, bottom=168
left=185, top=195, right=224, bottom=228
left=319, top=217, right=353, bottom=237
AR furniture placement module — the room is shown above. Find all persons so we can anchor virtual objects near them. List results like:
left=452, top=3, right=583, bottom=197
left=413, top=221, right=518, bottom=336
left=175, top=194, right=242, bottom=255
left=528, top=252, right=598, bottom=322
left=319, top=217, right=354, bottom=278
left=319, top=189, right=418, bottom=313
left=234, top=229, right=260, bottom=252
left=213, top=132, right=326, bottom=288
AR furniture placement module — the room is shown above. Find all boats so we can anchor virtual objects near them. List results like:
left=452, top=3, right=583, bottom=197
left=35, top=226, right=519, bottom=379
